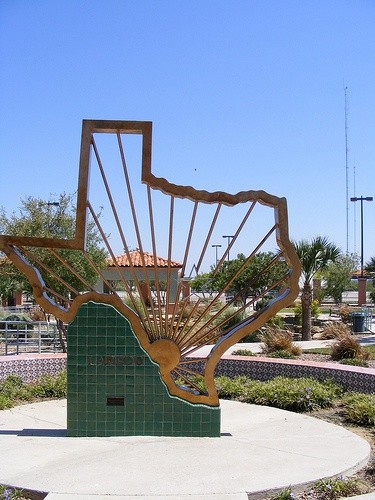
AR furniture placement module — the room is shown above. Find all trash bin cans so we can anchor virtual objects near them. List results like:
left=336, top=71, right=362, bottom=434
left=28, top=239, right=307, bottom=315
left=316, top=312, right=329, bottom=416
left=349, top=313, right=366, bottom=333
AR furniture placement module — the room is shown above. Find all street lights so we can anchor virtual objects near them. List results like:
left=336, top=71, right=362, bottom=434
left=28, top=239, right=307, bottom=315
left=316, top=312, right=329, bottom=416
left=350, top=196, right=372, bottom=274
left=221, top=235, right=235, bottom=261
left=211, top=245, right=222, bottom=268
